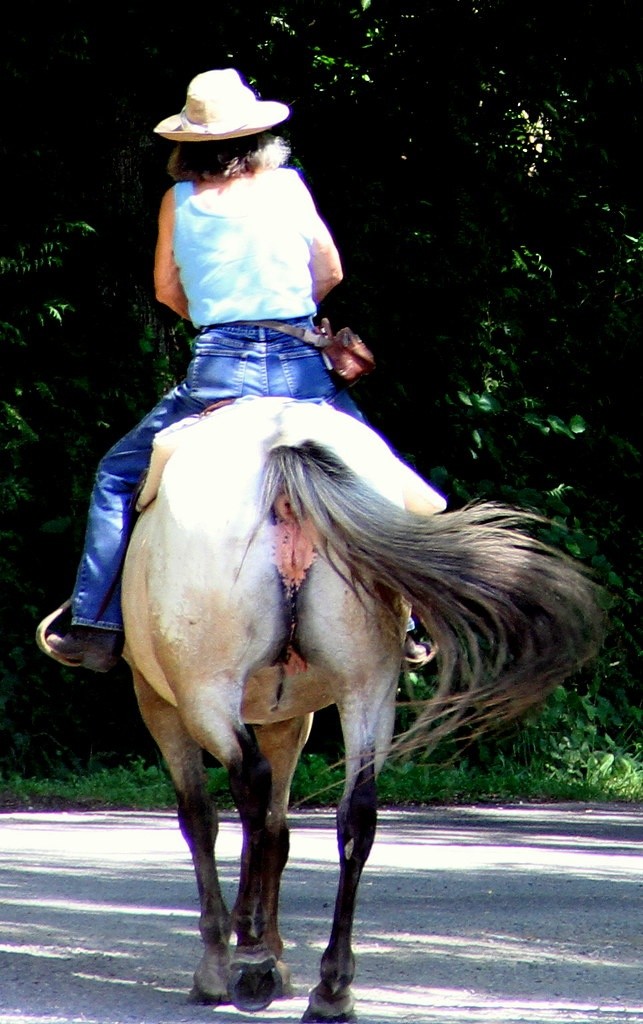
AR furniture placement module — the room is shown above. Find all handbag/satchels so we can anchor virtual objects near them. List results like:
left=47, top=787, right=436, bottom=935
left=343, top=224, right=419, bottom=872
left=234, top=320, right=375, bottom=388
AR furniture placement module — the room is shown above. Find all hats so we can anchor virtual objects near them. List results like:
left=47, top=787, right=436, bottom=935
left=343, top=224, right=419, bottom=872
left=152, top=68, right=289, bottom=142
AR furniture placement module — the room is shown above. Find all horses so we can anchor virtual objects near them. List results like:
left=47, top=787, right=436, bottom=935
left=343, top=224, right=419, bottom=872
left=120, top=395, right=603, bottom=1024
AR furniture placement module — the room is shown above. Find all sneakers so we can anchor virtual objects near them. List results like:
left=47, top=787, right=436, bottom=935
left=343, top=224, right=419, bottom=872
left=402, top=640, right=435, bottom=670
left=44, top=627, right=116, bottom=672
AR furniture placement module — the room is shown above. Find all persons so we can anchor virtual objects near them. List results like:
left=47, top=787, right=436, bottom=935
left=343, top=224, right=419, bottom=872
left=40, top=66, right=377, bottom=676
